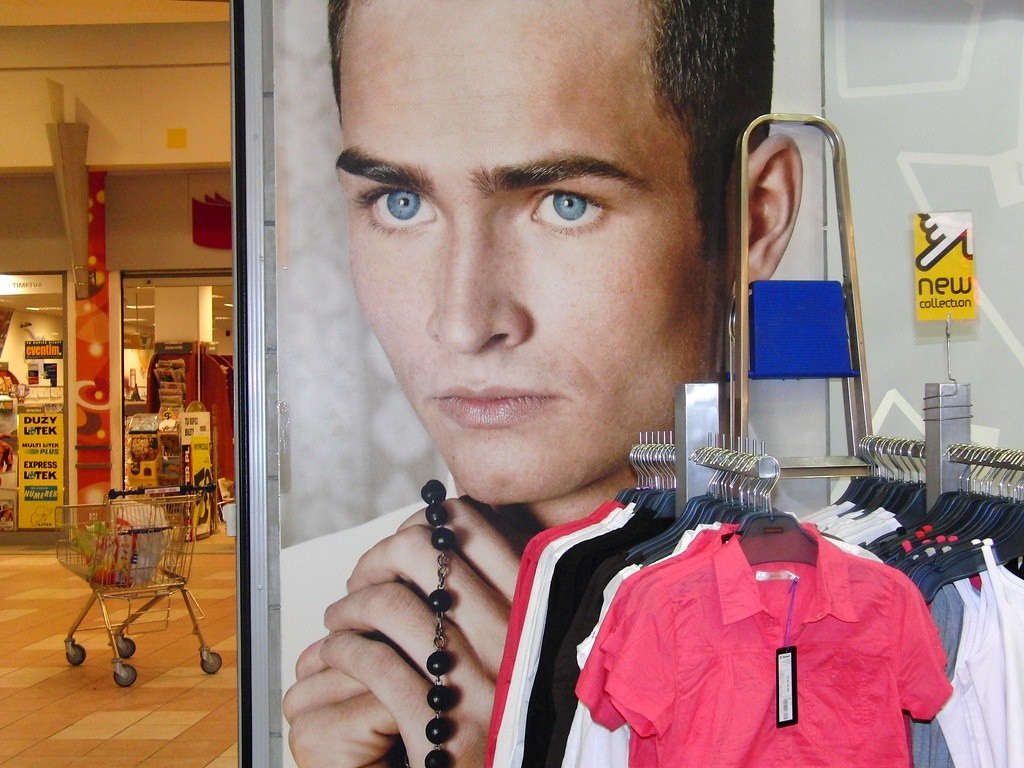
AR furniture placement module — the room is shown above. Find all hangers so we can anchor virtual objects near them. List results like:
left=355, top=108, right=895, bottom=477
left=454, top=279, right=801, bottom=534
left=614, top=442, right=819, bottom=572
left=836, top=437, right=1024, bottom=603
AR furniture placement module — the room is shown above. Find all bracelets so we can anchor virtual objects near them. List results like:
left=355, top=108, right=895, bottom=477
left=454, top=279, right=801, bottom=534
left=420, top=479, right=459, bottom=768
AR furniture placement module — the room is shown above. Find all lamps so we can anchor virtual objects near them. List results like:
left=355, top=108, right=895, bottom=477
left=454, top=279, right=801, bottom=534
left=45, top=123, right=89, bottom=301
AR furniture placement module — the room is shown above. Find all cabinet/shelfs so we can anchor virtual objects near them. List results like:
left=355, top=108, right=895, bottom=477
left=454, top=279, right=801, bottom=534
left=127, top=412, right=215, bottom=539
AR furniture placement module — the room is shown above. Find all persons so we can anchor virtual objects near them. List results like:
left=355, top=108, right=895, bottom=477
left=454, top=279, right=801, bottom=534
left=282, top=0, right=804, bottom=768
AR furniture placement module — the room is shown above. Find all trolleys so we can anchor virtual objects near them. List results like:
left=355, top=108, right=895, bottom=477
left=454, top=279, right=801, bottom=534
left=52, top=483, right=223, bottom=687
